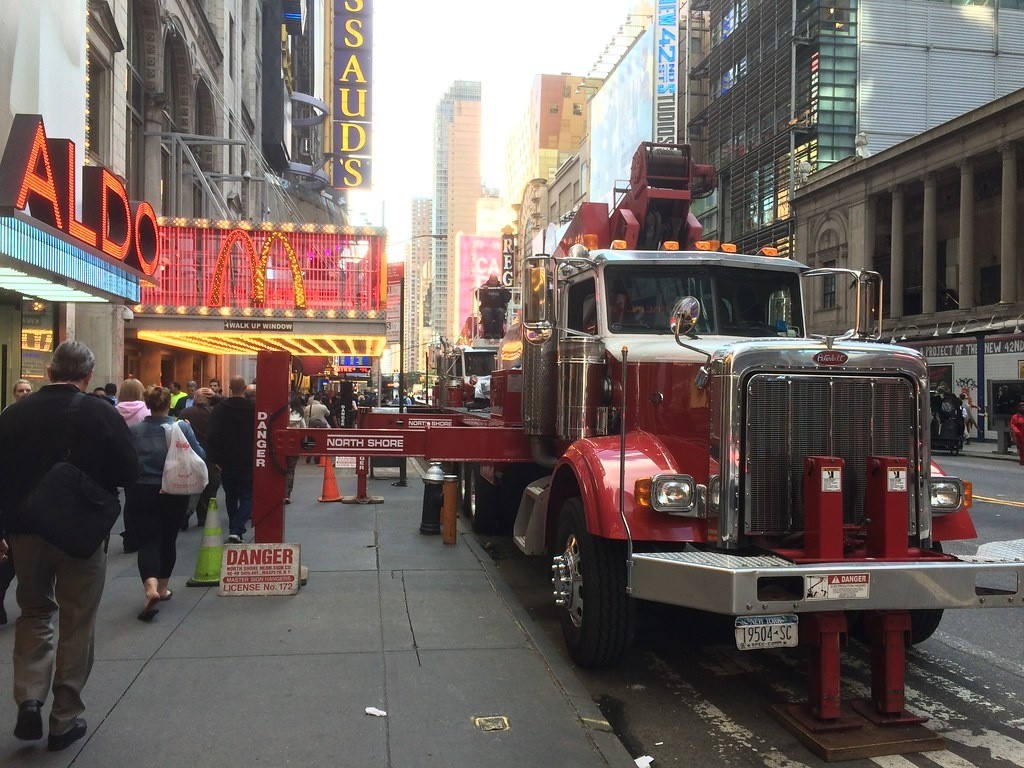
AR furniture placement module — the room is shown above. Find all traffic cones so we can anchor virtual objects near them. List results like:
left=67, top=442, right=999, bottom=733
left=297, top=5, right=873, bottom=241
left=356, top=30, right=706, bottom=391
left=315, top=455, right=328, bottom=468
left=185, top=498, right=223, bottom=588
left=314, top=454, right=344, bottom=502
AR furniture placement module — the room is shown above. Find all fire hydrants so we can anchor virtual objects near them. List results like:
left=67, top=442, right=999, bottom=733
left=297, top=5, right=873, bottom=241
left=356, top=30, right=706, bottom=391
left=418, top=461, right=446, bottom=535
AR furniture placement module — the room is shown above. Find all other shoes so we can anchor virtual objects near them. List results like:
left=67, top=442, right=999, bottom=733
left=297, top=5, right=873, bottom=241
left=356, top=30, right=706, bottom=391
left=228, top=532, right=244, bottom=543
left=14, top=701, right=44, bottom=740
left=306, top=457, right=311, bottom=463
left=285, top=498, right=291, bottom=504
left=48, top=718, right=87, bottom=750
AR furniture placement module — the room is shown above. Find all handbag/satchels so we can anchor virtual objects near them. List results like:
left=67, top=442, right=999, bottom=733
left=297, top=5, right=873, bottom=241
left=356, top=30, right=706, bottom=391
left=309, top=418, right=327, bottom=428
left=158, top=419, right=209, bottom=496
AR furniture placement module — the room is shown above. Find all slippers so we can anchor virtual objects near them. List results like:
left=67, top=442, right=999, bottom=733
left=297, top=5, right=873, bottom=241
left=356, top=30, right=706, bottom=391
left=137, top=609, right=159, bottom=620
left=160, top=588, right=172, bottom=600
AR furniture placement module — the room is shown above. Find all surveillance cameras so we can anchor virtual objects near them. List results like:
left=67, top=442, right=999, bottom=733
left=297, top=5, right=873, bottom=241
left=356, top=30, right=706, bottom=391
left=268, top=207, right=271, bottom=214
left=243, top=171, right=251, bottom=181
left=121, top=309, right=134, bottom=322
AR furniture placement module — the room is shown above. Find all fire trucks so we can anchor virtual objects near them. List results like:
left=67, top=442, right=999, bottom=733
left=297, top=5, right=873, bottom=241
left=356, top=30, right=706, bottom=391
left=252, top=141, right=1023, bottom=735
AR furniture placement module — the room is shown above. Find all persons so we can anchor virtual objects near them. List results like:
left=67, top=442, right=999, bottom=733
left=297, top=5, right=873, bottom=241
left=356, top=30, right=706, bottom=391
left=937, top=382, right=982, bottom=444
left=1010, top=402, right=1024, bottom=466
left=476, top=274, right=511, bottom=338
left=0, top=340, right=417, bottom=752
